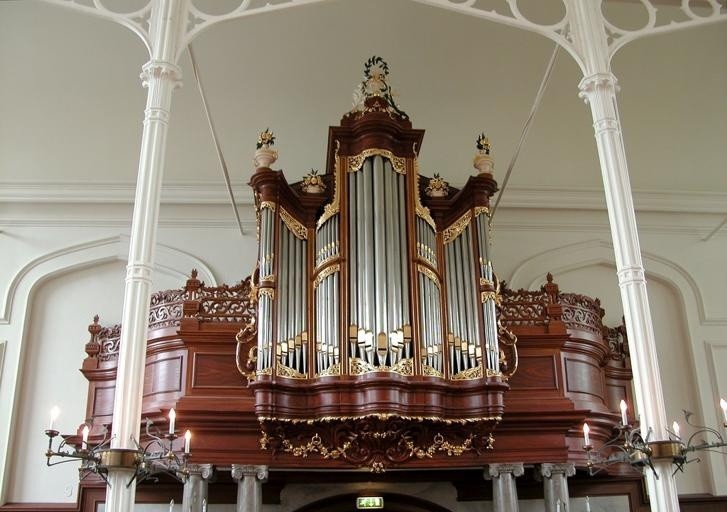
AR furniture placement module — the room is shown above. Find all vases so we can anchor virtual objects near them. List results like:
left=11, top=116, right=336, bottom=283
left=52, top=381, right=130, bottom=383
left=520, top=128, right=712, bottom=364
left=473, top=156, right=494, bottom=175
left=305, top=184, right=321, bottom=194
left=428, top=189, right=446, bottom=197
left=255, top=150, right=276, bottom=167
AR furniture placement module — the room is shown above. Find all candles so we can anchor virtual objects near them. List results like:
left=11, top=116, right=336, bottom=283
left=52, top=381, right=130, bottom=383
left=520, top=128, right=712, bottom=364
left=81, top=434, right=88, bottom=449
left=47, top=416, right=56, bottom=430
left=674, top=431, right=680, bottom=441
left=167, top=418, right=190, bottom=453
left=620, top=410, right=628, bottom=426
left=583, top=430, right=590, bottom=445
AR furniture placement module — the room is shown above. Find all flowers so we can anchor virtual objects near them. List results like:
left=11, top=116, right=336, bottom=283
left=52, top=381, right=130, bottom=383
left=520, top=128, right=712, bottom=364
left=473, top=130, right=492, bottom=158
left=300, top=168, right=326, bottom=191
left=255, top=127, right=278, bottom=154
left=424, top=170, right=449, bottom=192
